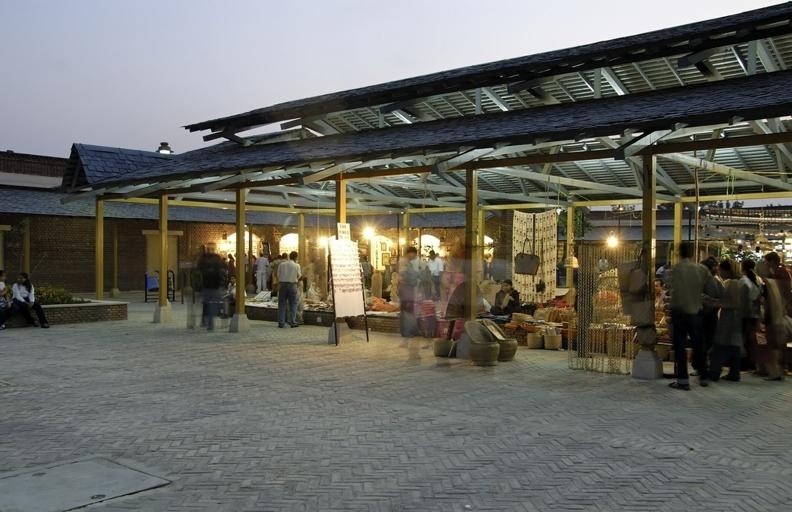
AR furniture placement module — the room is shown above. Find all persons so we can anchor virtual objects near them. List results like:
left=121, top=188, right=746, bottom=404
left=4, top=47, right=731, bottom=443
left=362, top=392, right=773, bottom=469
left=196, top=251, right=306, bottom=331
left=654, top=240, right=792, bottom=391
left=490, top=279, right=521, bottom=316
left=12, top=272, right=50, bottom=328
left=398, top=246, right=483, bottom=341
left=361, top=256, right=374, bottom=291
left=0, top=269, right=9, bottom=330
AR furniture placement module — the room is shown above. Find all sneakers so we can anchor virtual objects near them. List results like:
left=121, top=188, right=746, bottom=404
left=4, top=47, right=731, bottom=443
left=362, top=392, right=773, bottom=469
left=33, top=321, right=40, bottom=327
left=41, top=324, right=50, bottom=328
left=668, top=370, right=741, bottom=391
left=291, top=323, right=298, bottom=328
left=279, top=323, right=285, bottom=328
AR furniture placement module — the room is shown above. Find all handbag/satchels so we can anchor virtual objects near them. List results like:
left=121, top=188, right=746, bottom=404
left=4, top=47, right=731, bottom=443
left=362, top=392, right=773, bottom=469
left=514, top=252, right=540, bottom=276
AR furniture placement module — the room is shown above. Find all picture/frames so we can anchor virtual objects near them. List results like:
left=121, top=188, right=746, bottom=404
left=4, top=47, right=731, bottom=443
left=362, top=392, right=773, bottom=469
left=381, top=242, right=397, bottom=266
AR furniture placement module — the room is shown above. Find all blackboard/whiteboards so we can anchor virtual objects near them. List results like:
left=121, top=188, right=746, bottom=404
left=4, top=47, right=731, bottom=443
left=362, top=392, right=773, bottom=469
left=329, top=240, right=364, bottom=317
